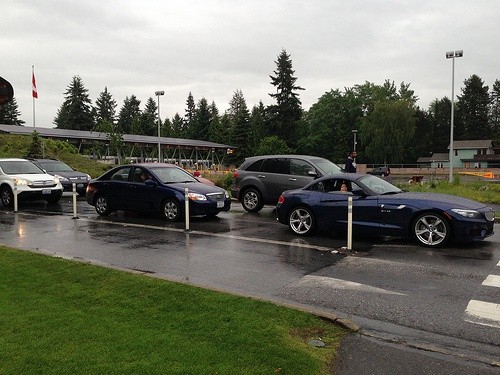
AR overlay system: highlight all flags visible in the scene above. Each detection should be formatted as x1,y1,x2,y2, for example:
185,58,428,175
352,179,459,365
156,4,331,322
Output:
32,73,38,98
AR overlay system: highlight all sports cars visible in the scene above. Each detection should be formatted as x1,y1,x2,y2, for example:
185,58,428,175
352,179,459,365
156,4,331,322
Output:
275,172,495,249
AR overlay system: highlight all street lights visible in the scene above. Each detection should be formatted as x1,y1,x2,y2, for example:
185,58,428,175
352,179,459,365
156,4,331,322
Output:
445,50,464,186
155,91,165,162
352,130,358,162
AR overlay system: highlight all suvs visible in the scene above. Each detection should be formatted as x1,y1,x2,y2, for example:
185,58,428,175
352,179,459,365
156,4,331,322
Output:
231,154,367,214
0,157,63,211
365,167,390,176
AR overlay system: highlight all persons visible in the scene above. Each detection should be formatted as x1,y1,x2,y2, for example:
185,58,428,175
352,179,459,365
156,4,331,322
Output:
345,151,358,173
340,183,347,192
140,172,148,183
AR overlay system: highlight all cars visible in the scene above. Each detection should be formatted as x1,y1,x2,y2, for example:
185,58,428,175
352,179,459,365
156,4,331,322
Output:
86,162,233,222
194,176,216,186
23,158,92,197
